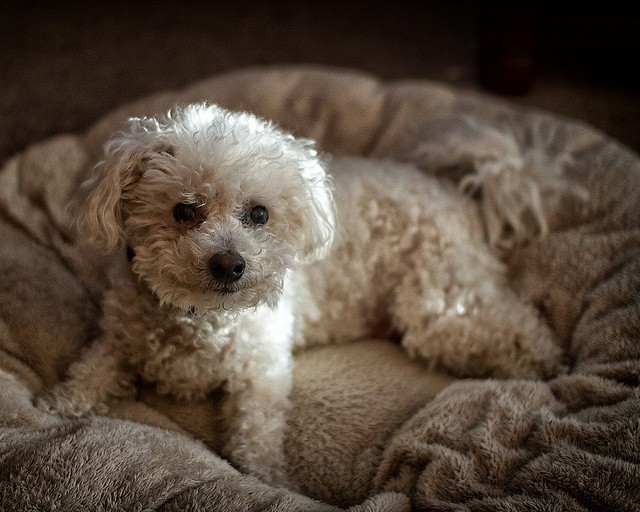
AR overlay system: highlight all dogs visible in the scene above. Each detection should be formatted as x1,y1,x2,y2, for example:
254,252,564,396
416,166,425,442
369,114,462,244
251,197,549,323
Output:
37,99,563,488
